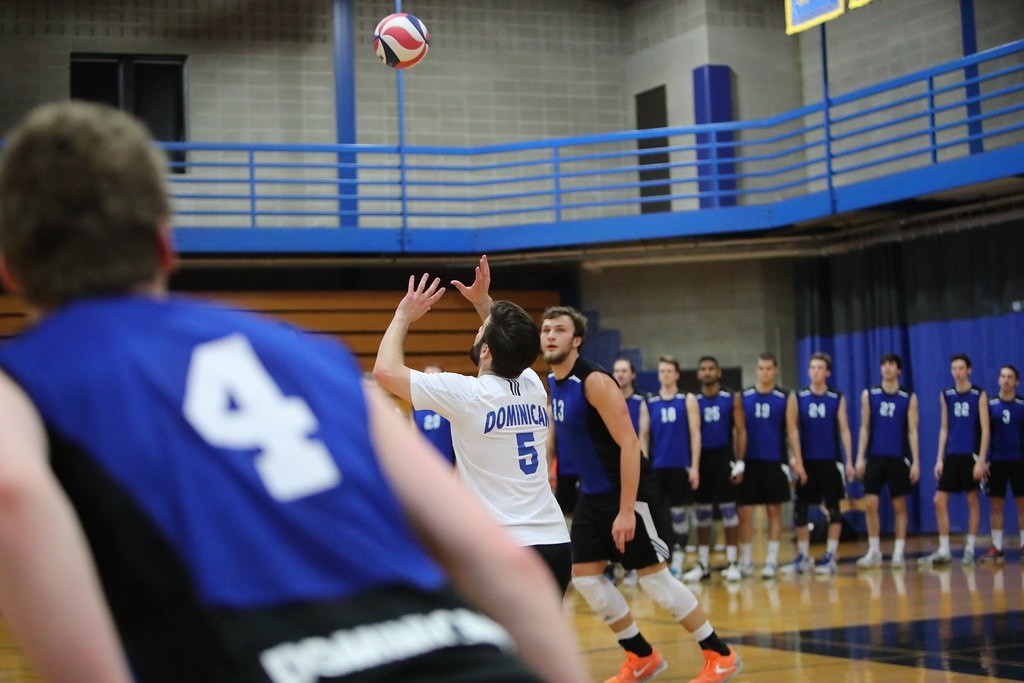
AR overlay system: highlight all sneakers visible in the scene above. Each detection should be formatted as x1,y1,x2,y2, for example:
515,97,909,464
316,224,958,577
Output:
891,550,905,567
960,550,976,566
686,643,743,683
980,545,1005,560
760,559,778,578
917,552,952,566
813,551,839,574
600,643,668,683
855,552,883,567
778,553,810,573
682,561,712,581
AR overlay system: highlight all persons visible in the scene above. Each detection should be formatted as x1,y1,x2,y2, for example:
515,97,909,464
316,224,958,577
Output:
597,561,1024,683
0,98,595,683
917,353,1024,565
371,255,573,610
413,366,456,465
540,306,744,683
603,351,855,588
855,353,921,568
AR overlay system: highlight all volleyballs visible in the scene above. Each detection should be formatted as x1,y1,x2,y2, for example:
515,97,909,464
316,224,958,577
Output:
373,12,431,70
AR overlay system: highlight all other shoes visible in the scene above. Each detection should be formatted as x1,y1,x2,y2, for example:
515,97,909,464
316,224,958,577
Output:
621,570,637,586
670,566,683,579
719,562,754,581
